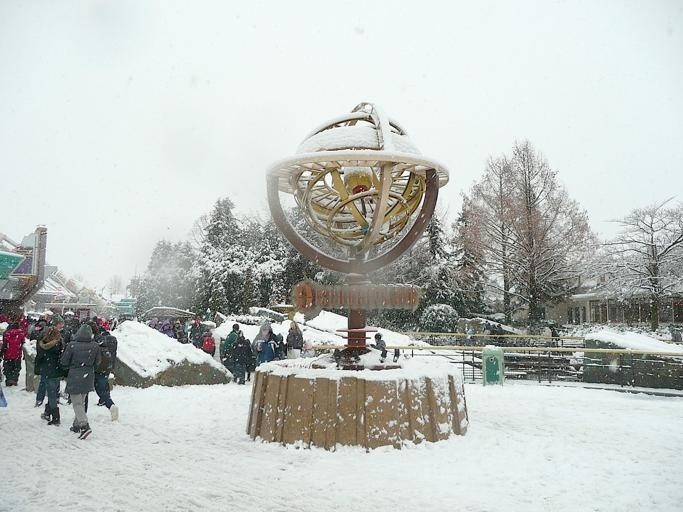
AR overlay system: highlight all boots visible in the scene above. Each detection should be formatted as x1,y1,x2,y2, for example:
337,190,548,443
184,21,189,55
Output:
40,404,60,425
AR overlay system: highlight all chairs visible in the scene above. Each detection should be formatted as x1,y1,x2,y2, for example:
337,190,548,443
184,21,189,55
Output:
461,343,585,385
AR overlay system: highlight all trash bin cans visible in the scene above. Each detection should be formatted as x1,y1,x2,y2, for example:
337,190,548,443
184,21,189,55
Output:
482,345,505,387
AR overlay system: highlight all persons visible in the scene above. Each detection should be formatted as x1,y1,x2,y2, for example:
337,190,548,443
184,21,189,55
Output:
33,327,65,427
483,320,560,346
58,324,102,440
222,321,303,384
51,314,122,344
147,317,216,358
370,333,385,351
0,310,51,392
91,322,118,421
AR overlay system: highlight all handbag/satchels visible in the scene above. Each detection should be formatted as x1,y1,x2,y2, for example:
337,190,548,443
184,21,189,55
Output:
203,336,214,353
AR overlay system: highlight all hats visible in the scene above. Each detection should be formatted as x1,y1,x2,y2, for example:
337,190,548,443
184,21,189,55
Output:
78,324,93,337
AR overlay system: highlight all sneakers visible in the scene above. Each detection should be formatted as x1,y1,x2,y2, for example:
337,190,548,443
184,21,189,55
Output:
111,406,119,421
70,424,92,440
